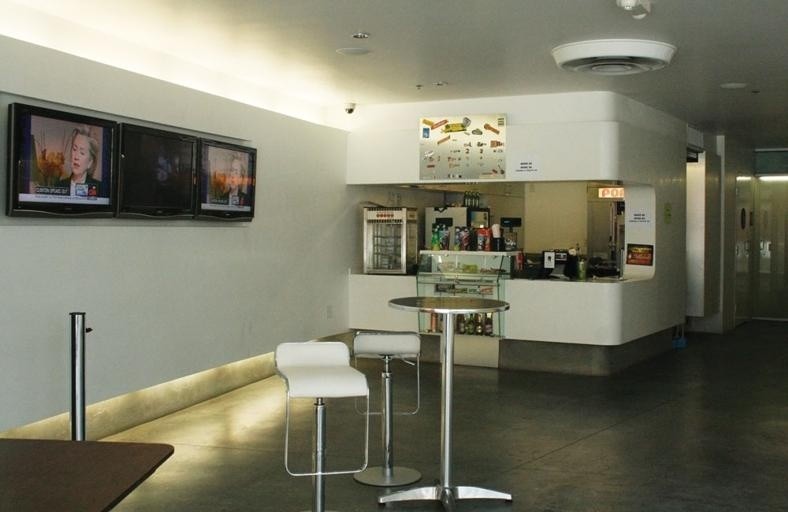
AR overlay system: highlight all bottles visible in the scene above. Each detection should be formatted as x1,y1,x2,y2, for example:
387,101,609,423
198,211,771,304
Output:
431,314,492,335
430,223,504,251
464,191,480,207
577,256,587,282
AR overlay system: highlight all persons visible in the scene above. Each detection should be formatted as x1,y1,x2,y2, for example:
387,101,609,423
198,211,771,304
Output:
53,124,103,198
219,152,251,207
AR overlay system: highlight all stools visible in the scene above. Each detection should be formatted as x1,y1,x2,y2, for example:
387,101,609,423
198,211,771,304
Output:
274,342,370,512
352,332,422,488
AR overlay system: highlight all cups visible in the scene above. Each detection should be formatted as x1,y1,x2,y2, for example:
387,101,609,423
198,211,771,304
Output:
504,232,517,251
511,253,524,276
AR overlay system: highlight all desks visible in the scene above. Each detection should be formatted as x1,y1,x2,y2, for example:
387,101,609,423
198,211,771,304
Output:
377,298,512,512
0,437,174,512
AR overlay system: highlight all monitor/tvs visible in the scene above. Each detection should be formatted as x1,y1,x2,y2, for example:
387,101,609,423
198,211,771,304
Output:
118,122,198,222
8,101,118,222
198,137,256,222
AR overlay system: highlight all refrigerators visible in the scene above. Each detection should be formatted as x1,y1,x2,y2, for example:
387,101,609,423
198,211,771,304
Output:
362,206,417,277
425,207,492,249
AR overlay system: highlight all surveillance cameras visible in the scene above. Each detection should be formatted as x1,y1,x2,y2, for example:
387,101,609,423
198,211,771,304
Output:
344,104,357,114
